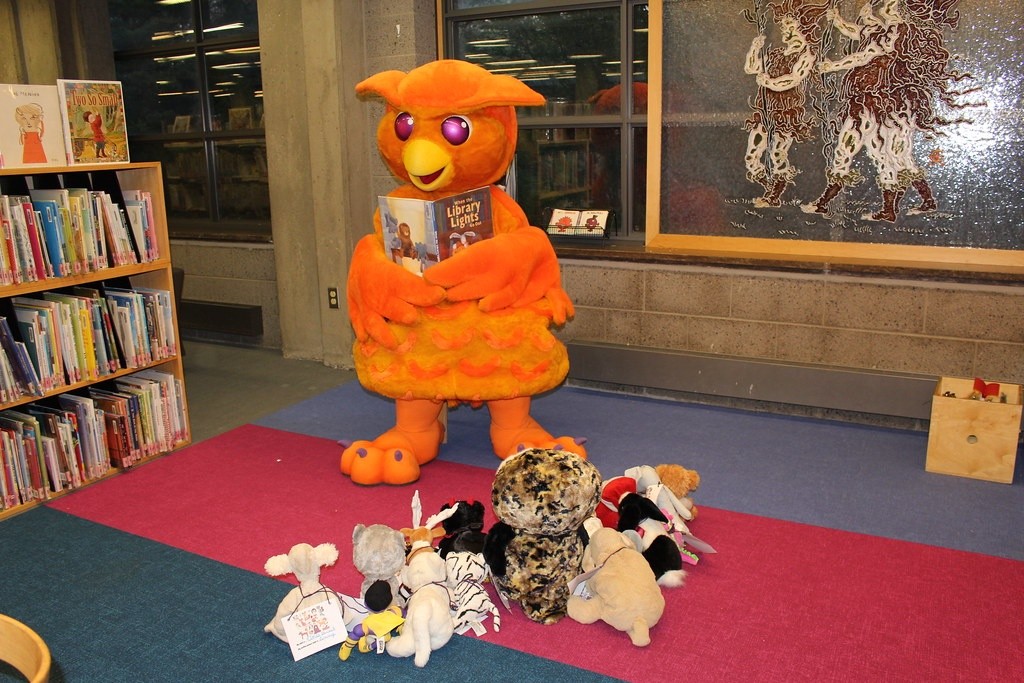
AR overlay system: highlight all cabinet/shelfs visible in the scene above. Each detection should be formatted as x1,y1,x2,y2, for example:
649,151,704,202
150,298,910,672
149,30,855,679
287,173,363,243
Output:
0,161,192,520
504,101,604,224
161,117,270,220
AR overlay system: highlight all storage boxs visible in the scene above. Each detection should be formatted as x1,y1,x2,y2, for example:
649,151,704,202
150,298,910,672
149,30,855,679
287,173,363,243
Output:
924,373,1023,485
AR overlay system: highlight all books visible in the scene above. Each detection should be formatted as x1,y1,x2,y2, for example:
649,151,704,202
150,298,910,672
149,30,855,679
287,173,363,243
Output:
375,183,494,278
0,178,161,291
0,283,179,407
54,77,132,165
0,366,191,517
576,210,609,237
547,208,580,238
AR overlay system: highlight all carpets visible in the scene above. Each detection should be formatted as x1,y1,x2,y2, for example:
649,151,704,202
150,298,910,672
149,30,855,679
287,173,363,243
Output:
0,375,1024,683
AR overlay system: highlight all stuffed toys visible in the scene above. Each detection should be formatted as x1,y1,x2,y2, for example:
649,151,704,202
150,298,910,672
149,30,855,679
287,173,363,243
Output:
263,447,722,669
337,53,590,488
